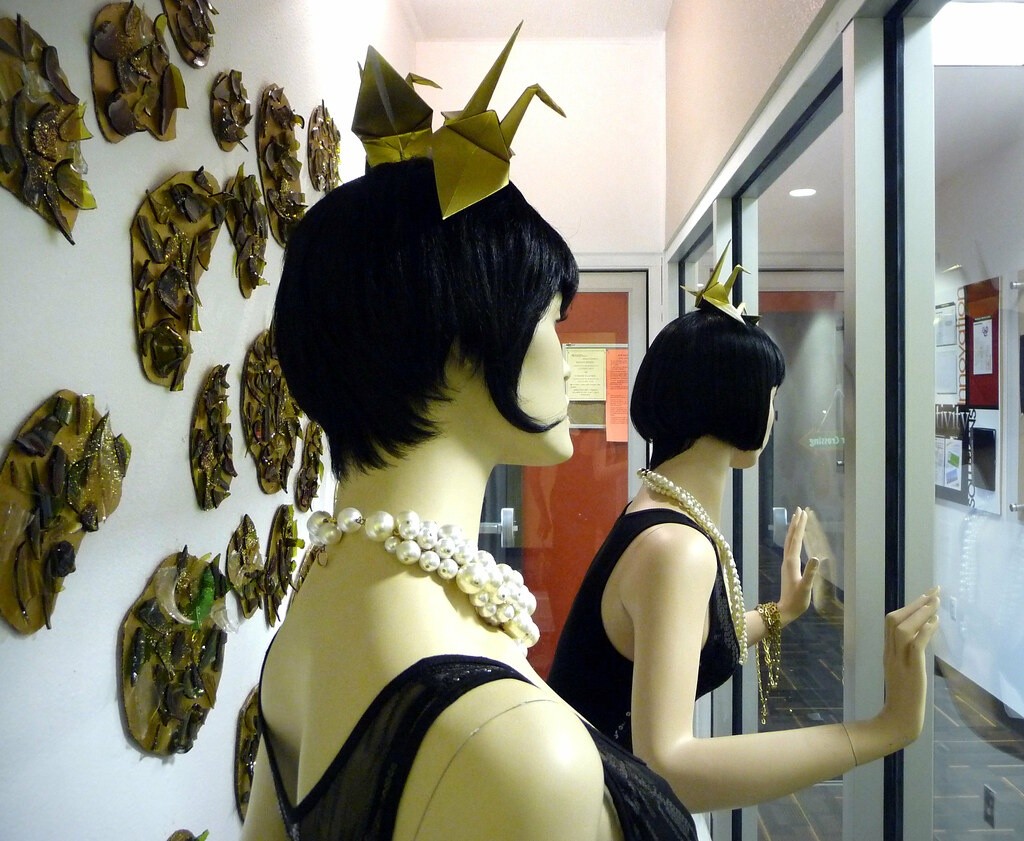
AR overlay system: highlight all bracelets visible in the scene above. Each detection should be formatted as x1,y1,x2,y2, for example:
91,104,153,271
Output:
749,605,781,727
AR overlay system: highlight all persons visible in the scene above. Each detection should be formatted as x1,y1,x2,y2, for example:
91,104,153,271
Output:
549,312,943,814
230,156,697,841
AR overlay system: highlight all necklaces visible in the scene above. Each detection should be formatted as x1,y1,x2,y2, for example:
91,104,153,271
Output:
637,467,748,665
303,509,539,647
